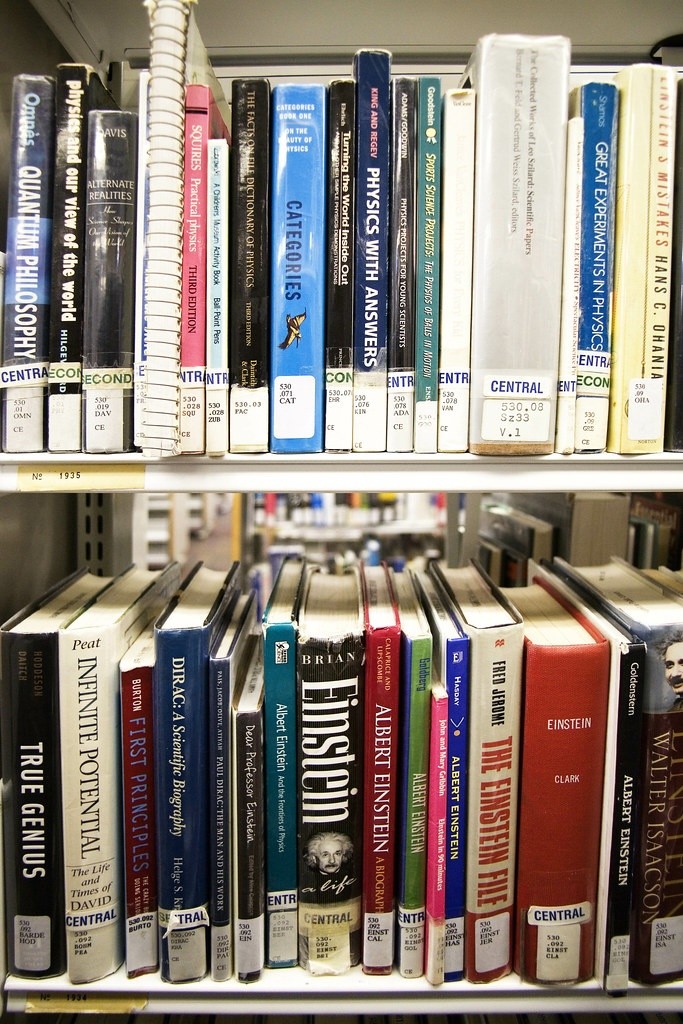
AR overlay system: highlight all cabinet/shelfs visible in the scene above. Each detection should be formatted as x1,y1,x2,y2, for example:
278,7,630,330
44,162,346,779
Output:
1,0,683,1024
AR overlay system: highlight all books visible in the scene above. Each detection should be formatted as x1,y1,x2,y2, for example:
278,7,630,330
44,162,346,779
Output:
0,0,683,1024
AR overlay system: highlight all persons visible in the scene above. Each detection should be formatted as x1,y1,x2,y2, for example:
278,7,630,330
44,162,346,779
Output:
655,630,683,713
303,831,354,903
